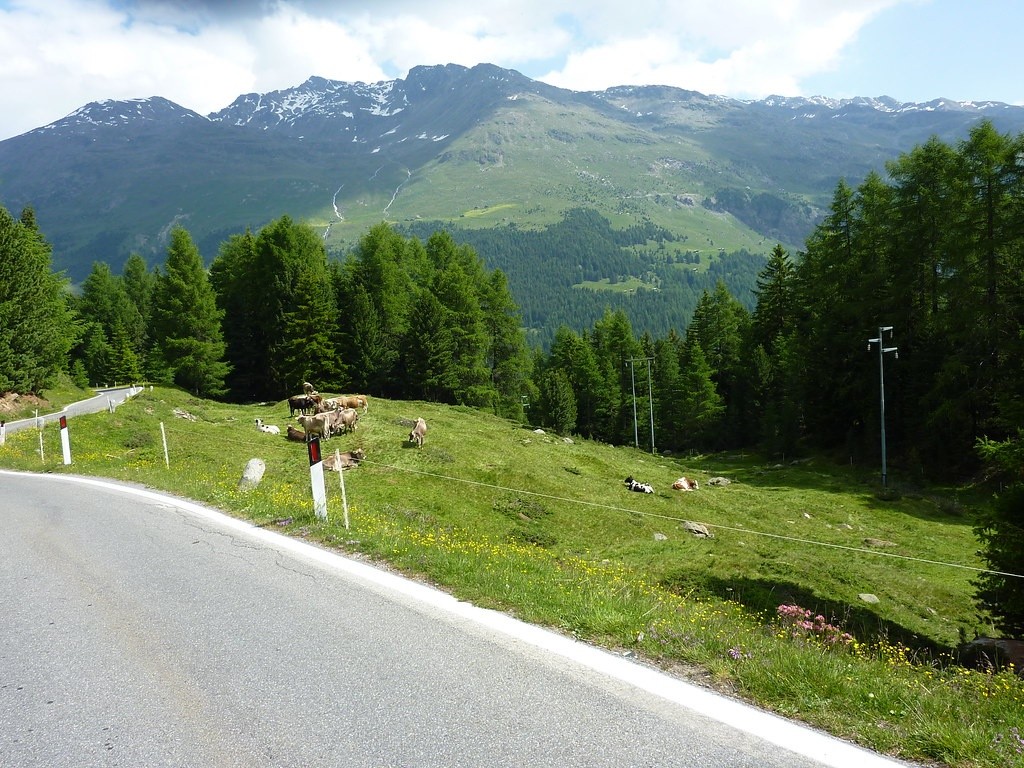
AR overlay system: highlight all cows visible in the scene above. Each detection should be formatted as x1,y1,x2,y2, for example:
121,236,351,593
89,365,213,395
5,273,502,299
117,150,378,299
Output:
322,447,367,472
284,381,368,443
254,418,281,435
671,477,699,491
624,475,655,494
408,417,427,448
